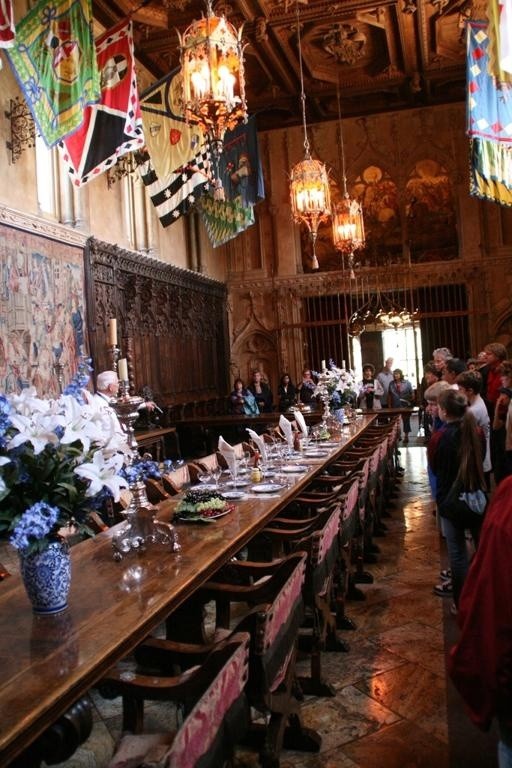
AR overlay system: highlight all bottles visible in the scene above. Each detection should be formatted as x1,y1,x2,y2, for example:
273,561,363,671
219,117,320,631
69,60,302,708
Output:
310,426,320,441
294,432,300,451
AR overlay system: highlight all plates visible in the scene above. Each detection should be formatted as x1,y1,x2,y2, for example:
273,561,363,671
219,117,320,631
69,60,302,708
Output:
179,508,231,522
190,440,338,500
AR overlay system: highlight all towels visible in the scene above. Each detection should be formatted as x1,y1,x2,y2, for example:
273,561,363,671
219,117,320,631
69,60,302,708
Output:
217,406,313,481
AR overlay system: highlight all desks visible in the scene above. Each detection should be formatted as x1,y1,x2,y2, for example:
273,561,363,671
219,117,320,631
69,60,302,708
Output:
131,427,176,461
168,406,421,460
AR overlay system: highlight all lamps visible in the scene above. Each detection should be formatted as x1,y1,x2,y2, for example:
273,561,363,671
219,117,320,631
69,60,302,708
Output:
172,1,251,200
328,80,370,282
288,12,336,272
347,291,422,339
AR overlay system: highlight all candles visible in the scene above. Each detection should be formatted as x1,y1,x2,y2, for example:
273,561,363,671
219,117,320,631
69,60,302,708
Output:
117,357,129,382
107,318,118,347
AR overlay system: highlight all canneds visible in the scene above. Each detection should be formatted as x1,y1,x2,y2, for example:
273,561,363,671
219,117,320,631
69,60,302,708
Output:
250,468,260,482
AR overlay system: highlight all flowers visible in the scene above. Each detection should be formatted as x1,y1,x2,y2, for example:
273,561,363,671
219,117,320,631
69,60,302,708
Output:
1,381,134,546
305,368,360,406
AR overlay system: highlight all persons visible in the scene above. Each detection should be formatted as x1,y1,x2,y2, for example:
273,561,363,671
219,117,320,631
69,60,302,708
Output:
447,472,512,768
230,368,320,416
417,342,511,594
93,366,160,467
356,358,415,444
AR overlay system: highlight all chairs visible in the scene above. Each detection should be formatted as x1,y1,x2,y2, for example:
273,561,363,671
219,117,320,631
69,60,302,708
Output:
110,479,172,522
161,463,207,494
52,507,108,542
192,416,404,679
137,551,323,765
87,631,251,768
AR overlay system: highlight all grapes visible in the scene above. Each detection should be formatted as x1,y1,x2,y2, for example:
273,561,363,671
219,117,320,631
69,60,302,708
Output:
172,487,236,519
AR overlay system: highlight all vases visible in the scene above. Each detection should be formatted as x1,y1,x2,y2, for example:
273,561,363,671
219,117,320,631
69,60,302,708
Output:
17,537,74,616
330,401,344,429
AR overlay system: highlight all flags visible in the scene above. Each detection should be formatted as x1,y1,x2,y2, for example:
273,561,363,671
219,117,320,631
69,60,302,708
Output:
0,0,18,48
127,66,224,230
57,11,148,191
192,79,265,250
7,0,104,147
462,17,511,208
